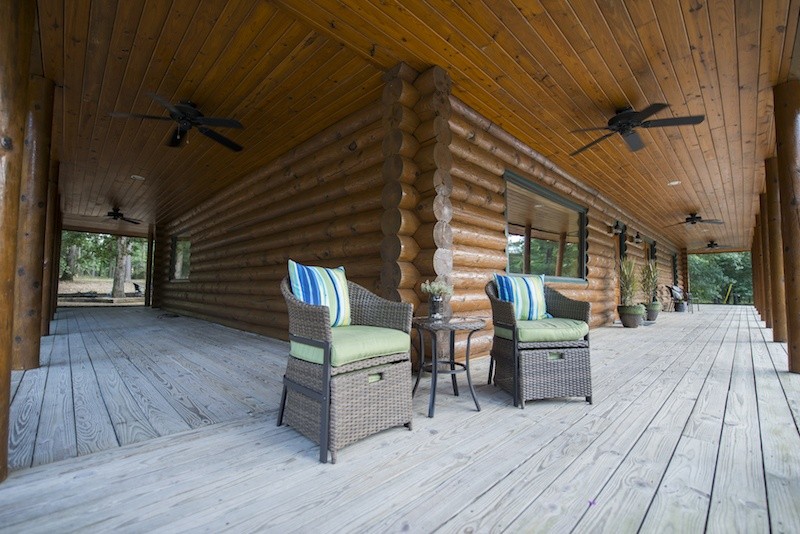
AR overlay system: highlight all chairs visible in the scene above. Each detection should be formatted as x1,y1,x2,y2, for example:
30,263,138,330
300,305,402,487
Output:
277,274,414,463
666,285,699,314
486,280,593,408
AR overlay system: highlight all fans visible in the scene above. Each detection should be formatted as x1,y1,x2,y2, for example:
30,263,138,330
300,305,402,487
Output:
104,208,142,224
664,212,725,228
110,89,245,152
698,240,735,249
569,103,706,156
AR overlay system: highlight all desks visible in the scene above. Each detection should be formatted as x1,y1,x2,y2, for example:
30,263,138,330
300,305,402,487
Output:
411,316,487,417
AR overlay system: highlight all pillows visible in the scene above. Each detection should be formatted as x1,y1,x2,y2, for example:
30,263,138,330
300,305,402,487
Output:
493,272,553,321
288,259,351,326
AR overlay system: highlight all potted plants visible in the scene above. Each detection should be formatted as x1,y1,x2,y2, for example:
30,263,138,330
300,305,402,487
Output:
615,255,662,328
422,280,454,318
